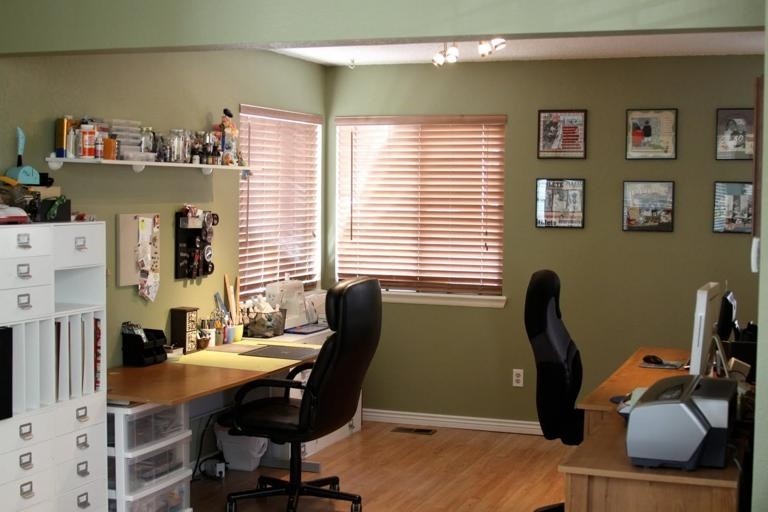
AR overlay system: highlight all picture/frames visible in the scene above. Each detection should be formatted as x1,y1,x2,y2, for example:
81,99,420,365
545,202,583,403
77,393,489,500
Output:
713,181,754,234
622,180,674,233
535,178,585,230
716,108,754,160
625,108,677,160
538,110,586,160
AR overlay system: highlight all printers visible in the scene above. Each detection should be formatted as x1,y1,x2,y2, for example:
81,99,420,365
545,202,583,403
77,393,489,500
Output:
627,374,737,471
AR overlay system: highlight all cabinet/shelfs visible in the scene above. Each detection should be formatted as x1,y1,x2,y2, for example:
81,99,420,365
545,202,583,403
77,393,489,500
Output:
0,222,108,512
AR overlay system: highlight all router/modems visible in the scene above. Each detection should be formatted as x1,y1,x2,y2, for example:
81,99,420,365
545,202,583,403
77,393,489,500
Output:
726,356,751,380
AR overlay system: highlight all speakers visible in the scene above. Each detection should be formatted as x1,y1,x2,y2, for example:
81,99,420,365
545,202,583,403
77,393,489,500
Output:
717,341,756,382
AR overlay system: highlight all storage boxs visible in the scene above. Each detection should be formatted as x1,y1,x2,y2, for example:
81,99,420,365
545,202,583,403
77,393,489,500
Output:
106,403,193,511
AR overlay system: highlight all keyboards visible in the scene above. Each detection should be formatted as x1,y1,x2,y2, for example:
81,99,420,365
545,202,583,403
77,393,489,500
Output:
617,386,648,420
684,357,691,368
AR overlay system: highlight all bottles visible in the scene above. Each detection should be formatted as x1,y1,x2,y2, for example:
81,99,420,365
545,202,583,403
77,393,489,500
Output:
67,118,118,160
142,126,222,165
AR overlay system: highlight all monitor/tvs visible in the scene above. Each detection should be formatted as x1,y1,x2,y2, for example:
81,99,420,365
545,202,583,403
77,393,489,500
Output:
717,289,741,341
689,282,732,378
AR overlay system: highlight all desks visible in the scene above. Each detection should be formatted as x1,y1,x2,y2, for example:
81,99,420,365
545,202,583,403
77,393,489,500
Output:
557,422,741,512
575,347,754,421
109,313,326,404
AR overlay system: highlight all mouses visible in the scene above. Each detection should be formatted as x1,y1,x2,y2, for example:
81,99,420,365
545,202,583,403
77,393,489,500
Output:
643,353,663,365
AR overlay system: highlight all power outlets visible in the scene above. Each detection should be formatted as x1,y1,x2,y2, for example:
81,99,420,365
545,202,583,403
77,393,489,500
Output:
512,368,524,388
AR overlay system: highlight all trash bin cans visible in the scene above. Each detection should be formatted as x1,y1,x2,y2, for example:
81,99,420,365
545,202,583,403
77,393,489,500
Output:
213,424,268,472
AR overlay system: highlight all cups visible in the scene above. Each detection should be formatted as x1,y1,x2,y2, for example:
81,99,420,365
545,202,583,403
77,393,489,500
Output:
233,324,244,341
224,325,234,343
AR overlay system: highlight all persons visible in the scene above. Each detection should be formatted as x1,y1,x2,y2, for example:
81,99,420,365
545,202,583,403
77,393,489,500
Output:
723,118,746,152
642,121,652,147
544,113,562,151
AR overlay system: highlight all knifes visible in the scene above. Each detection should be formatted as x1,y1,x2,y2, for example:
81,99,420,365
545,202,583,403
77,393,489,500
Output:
16,127,25,166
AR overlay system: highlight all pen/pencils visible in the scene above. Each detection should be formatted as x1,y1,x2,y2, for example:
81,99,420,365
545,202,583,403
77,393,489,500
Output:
223,312,235,327
210,310,223,328
202,320,213,328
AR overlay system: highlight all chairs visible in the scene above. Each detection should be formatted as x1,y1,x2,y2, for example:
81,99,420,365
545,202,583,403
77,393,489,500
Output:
213,277,383,512
523,269,584,512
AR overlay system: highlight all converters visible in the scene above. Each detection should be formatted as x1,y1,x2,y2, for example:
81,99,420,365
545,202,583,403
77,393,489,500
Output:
206,462,225,478
